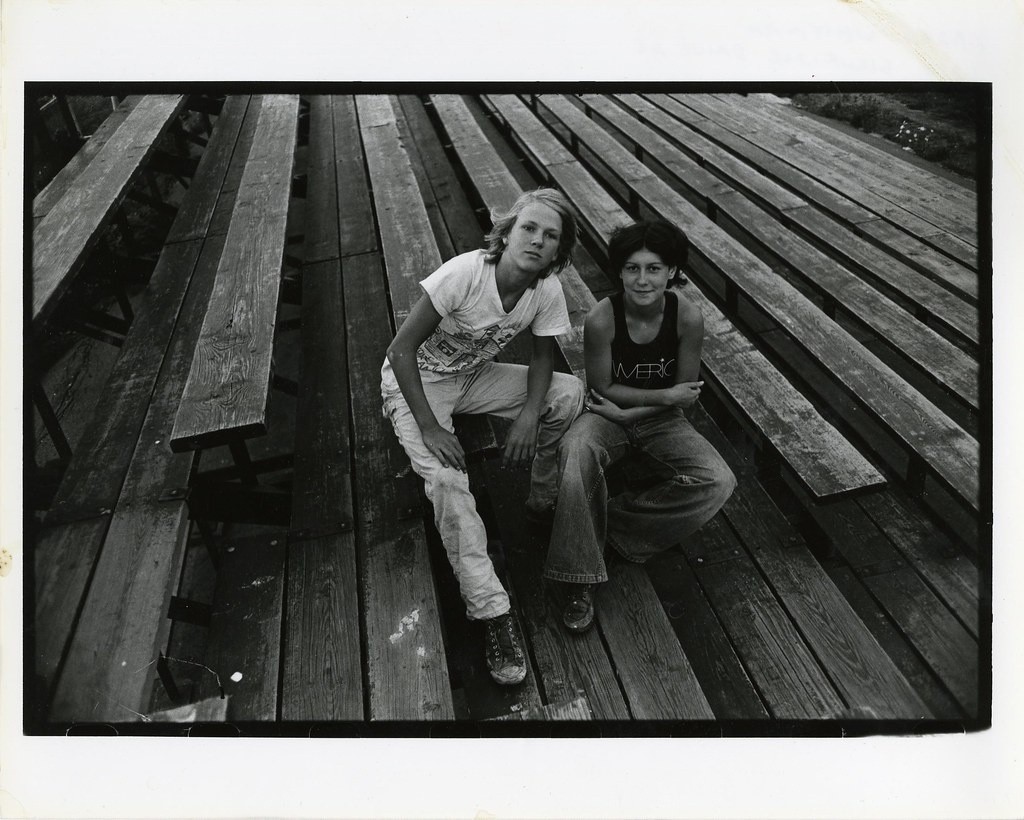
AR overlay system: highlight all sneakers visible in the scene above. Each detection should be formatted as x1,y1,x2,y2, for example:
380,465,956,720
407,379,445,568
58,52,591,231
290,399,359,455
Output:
603,545,625,571
563,579,596,632
485,616,527,685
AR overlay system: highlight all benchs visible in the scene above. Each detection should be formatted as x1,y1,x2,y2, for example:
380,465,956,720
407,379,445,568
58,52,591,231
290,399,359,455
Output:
28,92,979,718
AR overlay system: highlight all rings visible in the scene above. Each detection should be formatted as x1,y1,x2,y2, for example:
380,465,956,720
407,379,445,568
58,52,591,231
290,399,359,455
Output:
586,403,592,410
598,396,604,401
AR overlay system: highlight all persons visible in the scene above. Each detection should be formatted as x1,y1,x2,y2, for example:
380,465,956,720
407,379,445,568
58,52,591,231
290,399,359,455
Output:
544,217,739,637
380,188,585,686
